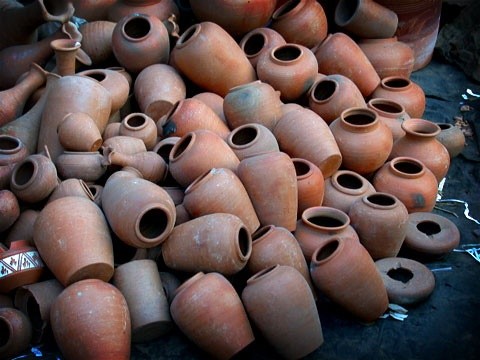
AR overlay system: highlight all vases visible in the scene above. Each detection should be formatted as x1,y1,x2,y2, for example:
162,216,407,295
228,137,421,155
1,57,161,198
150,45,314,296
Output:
0,0,465,360
